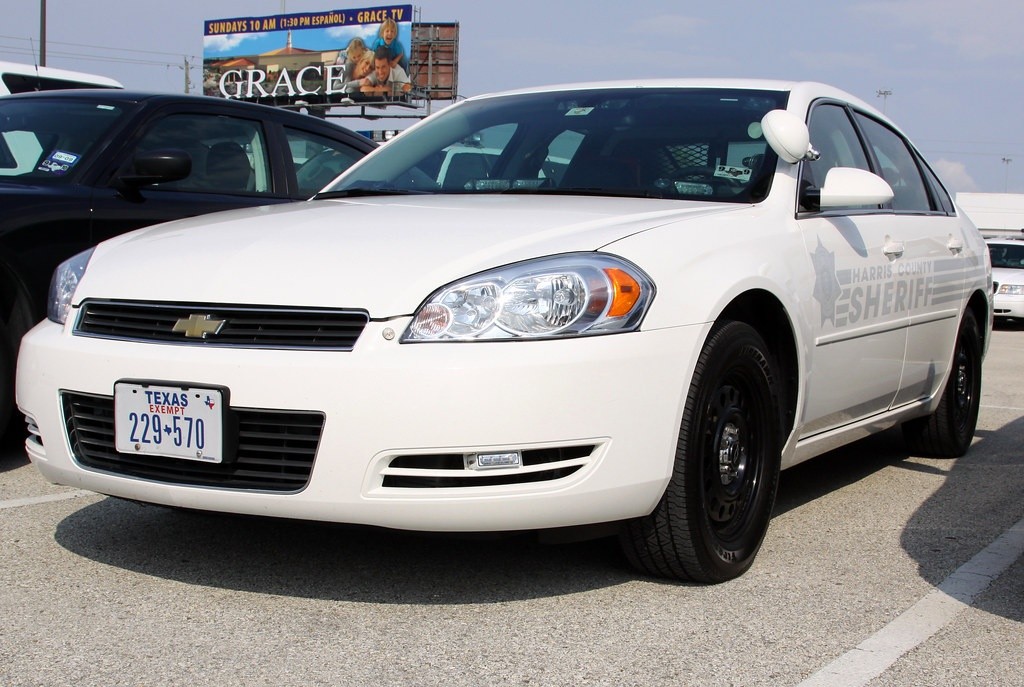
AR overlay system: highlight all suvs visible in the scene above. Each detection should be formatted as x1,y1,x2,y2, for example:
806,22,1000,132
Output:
0,59,126,179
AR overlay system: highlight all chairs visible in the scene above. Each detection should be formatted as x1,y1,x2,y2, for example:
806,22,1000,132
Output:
610,138,682,194
991,250,1002,261
206,142,251,191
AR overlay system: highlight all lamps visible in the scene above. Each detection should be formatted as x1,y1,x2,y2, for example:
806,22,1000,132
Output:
341,98,354,103
295,100,308,106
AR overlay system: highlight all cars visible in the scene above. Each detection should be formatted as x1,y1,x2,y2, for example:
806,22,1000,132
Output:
292,139,573,196
980,235,1024,318
0,88,444,436
15,76,995,586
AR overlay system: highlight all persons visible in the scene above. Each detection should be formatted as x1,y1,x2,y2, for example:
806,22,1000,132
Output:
330,17,412,103
